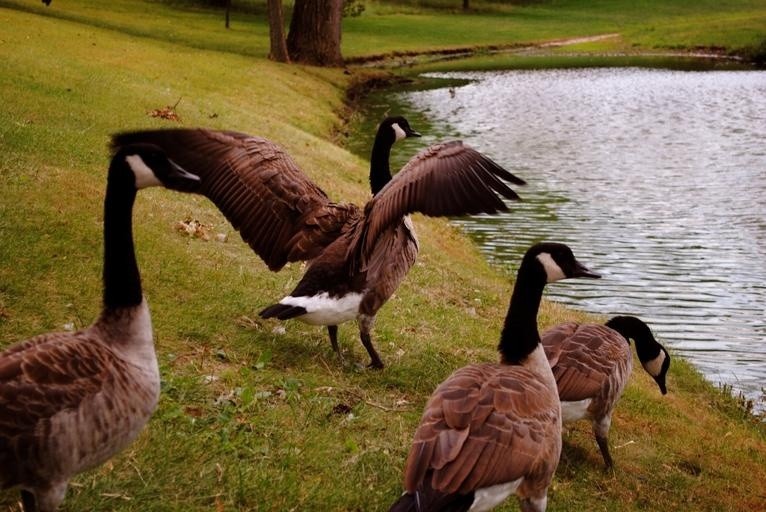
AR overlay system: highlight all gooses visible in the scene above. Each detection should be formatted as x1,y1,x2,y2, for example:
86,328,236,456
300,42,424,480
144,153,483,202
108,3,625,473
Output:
387,241,603,512
539,315,671,477
0,143,202,512
106,114,528,371
